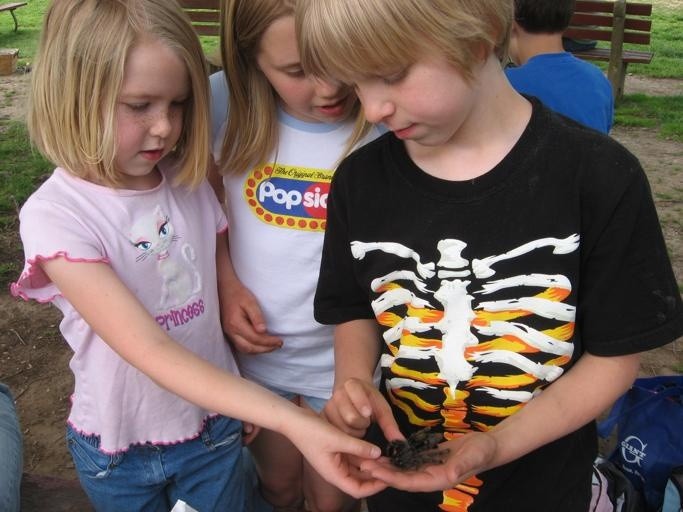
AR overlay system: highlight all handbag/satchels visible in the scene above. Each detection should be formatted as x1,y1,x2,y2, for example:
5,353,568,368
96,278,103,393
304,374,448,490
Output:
588,376,683,511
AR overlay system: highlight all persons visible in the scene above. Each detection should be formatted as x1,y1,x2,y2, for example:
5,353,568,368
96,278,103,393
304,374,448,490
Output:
8,0,390,512
215,0,421,512
499,0,615,136
294,0,682,512
